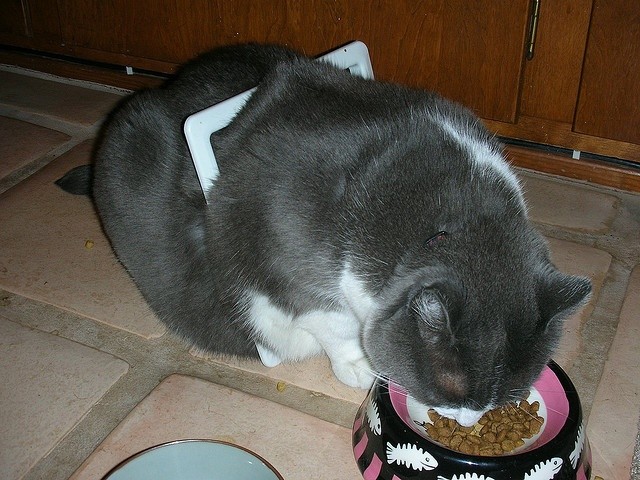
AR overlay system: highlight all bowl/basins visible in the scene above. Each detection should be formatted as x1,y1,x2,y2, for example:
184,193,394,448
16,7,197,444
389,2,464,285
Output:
351,357,592,480
98,438,285,480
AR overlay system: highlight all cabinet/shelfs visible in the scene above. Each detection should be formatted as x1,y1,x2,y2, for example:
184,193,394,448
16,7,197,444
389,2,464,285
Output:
574,1,639,193
2,1,542,171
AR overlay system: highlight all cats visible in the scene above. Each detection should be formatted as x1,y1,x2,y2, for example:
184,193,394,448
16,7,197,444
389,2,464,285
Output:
52,41,596,427
